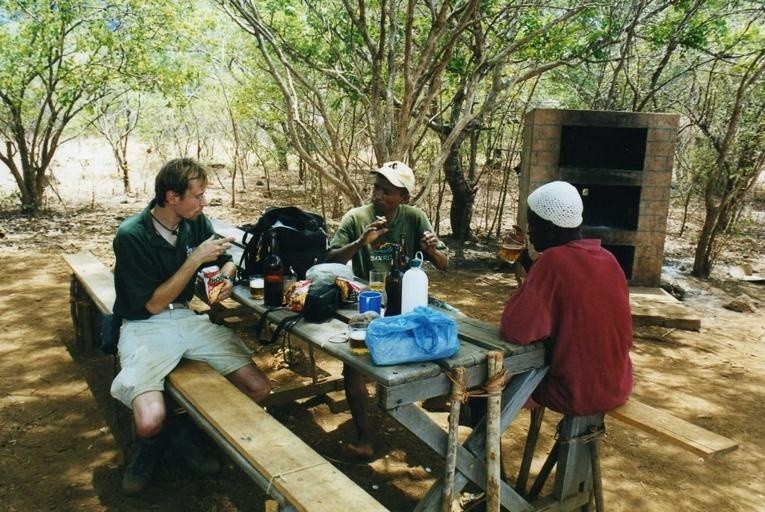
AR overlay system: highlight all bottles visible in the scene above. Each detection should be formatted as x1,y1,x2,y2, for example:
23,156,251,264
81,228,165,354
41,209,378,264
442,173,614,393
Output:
384,243,404,317
261,232,283,307
390,233,410,275
401,251,428,315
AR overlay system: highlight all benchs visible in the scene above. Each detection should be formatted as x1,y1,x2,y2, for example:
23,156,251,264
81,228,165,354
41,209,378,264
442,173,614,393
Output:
61,252,393,512
552,387,734,504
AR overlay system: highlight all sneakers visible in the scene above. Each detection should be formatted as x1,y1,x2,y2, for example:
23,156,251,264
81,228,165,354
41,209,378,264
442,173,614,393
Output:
121,440,160,497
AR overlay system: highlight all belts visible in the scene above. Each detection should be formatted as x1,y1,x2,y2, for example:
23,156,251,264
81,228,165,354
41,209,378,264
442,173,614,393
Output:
166,300,189,309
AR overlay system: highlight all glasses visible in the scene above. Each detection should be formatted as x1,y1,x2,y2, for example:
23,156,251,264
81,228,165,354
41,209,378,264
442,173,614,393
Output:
187,194,205,202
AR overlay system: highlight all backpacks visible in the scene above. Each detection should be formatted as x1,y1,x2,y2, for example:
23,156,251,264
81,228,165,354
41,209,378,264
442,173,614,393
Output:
230,206,329,285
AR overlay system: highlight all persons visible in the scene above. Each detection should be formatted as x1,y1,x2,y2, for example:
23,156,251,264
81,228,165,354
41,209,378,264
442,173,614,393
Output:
449,181,632,434
111,157,272,495
325,162,450,466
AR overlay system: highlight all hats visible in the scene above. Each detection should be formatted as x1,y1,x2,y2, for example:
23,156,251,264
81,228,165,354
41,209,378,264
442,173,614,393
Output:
527,181,584,228
369,160,416,194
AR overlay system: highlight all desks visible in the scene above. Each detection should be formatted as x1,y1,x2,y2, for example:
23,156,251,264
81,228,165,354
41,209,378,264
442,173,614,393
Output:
184,212,552,512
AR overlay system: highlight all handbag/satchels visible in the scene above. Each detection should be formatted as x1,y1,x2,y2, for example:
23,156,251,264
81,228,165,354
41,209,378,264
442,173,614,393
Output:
254,286,340,346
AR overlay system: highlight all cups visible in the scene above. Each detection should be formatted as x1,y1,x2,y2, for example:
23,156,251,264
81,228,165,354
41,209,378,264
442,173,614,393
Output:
498,233,526,265
349,322,371,354
359,293,381,318
283,273,297,296
369,270,387,294
248,274,266,300
